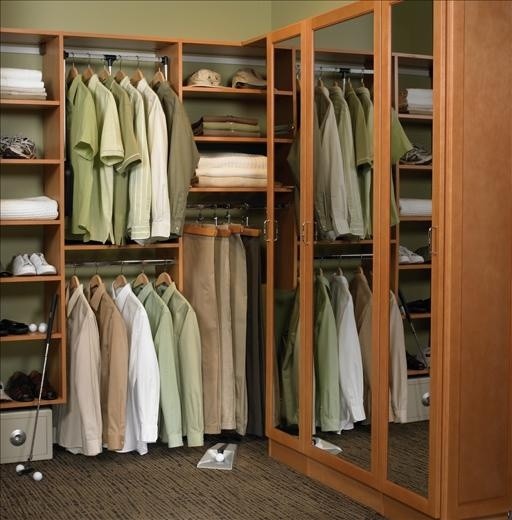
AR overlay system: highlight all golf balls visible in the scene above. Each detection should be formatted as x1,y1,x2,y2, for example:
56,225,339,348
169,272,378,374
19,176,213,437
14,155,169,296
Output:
27,322,49,334
215,454,224,460
14,465,26,474
33,472,42,481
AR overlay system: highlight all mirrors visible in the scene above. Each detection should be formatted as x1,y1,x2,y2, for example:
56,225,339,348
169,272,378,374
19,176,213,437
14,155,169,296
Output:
311,7,375,475
270,32,301,441
386,0,434,502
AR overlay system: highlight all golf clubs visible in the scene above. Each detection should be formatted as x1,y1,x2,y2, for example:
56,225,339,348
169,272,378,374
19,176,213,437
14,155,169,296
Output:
394,284,429,406
18,293,58,475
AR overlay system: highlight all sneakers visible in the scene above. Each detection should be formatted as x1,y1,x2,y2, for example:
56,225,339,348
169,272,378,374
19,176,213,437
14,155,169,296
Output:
403,246,424,264
4,371,34,402
0,321,8,337
399,245,411,265
406,299,429,313
28,370,57,400
29,252,57,275
12,253,37,276
406,350,424,370
1,319,29,335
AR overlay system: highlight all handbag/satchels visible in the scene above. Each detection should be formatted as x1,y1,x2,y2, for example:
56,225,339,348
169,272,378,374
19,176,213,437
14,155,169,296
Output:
0,136,37,160
399,142,433,165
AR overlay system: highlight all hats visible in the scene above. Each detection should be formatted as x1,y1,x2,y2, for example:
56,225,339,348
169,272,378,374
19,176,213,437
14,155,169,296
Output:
232,68,267,88
187,69,224,88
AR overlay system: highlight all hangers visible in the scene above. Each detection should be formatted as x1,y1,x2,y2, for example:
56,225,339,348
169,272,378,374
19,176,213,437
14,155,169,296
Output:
66,257,172,291
183,201,262,240
296,64,366,90
316,252,374,278
66,50,166,87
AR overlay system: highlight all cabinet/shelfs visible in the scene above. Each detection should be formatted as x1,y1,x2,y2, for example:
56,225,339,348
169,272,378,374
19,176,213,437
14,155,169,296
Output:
273,44,433,379
0,26,267,412
